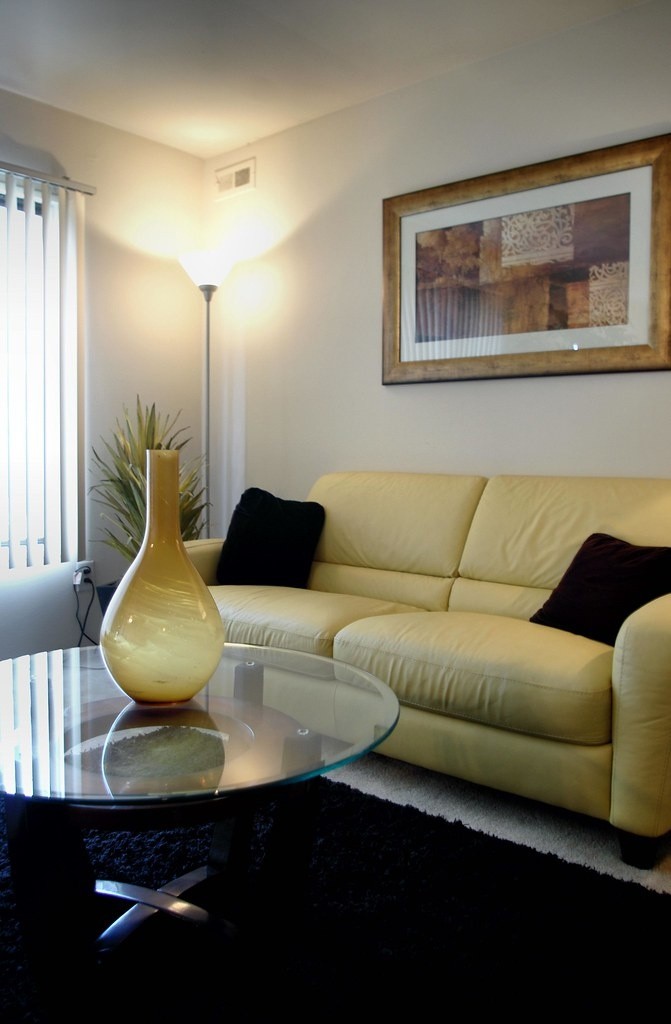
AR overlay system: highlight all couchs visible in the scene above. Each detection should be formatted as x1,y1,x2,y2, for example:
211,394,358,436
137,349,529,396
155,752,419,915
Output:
182,471,671,869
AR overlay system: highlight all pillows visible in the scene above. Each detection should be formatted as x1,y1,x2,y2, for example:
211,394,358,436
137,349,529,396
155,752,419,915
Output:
529,533,671,647
216,488,325,590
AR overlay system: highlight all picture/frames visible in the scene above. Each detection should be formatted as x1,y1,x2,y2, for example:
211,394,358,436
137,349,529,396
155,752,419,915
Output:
381,132,670,386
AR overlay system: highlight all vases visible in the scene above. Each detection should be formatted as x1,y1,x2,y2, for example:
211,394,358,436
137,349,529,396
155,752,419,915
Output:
98,448,224,704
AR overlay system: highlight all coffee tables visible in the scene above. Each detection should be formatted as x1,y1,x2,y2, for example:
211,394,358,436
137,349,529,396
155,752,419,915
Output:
0,642,399,953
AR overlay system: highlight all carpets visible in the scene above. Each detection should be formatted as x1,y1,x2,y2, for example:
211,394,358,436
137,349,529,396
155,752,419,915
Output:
319,750,671,894
0,725,671,1024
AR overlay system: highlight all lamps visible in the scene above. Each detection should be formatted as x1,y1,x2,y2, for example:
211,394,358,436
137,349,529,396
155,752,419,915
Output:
176,253,234,538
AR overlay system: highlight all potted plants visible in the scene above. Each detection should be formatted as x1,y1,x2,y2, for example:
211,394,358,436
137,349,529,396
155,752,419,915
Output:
85,393,213,617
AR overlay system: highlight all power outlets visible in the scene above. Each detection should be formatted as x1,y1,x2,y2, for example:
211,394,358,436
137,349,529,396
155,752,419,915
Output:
75,561,97,593
